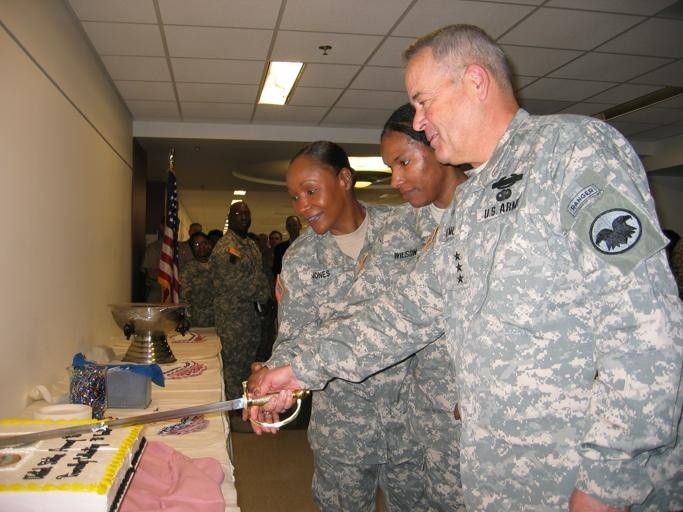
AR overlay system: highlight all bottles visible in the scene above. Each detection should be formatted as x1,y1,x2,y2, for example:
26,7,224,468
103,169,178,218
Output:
254,302,270,315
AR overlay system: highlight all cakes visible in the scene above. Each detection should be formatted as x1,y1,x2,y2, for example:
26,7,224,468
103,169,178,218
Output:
0,418,147,512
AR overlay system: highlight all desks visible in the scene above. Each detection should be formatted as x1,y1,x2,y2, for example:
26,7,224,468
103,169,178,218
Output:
0,319,237,511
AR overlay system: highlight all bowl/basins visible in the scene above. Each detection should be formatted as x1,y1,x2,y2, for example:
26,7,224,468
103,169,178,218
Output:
108,304,188,337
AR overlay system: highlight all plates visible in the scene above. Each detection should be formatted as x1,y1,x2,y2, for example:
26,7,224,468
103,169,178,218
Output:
33,403,93,421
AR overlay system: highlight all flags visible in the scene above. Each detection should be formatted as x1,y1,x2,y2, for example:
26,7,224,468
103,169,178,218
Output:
155,164,182,303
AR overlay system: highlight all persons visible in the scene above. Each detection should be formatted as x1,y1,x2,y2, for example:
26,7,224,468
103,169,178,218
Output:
248,99,473,512
255,24,682,512
243,141,426,512
141,201,303,417
